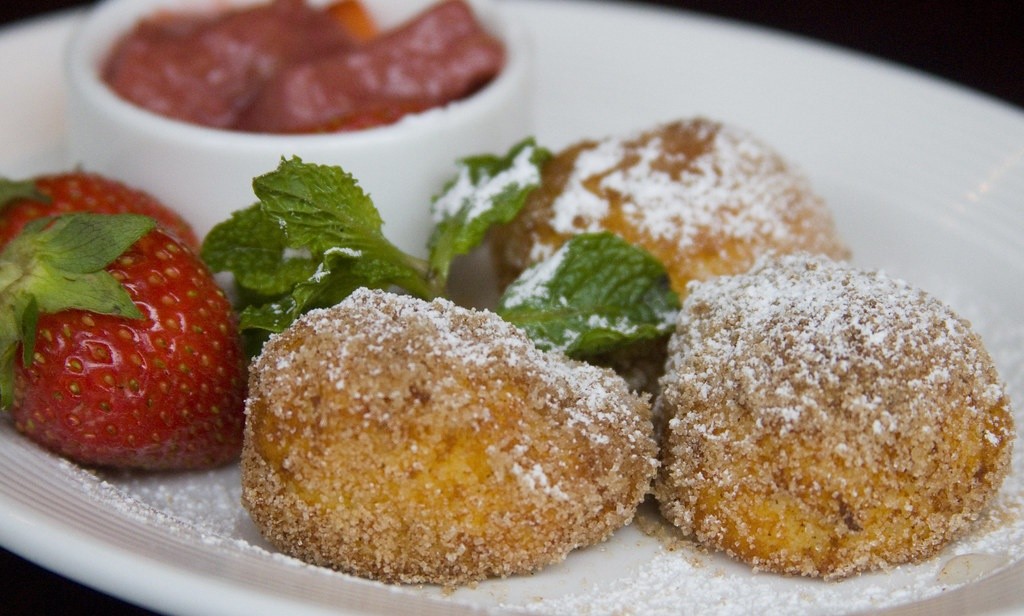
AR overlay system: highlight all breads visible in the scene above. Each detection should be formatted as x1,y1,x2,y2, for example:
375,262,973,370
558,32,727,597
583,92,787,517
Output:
239,285,659,586
485,114,853,310
641,257,1014,582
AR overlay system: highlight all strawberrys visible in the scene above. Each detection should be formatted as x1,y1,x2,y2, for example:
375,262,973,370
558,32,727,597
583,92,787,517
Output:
0,172,249,474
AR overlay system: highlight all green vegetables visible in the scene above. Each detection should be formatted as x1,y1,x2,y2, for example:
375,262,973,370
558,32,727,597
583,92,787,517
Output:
188,132,685,360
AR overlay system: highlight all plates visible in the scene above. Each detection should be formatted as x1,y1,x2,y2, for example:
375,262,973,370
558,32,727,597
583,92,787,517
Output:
0,1,1024,615
